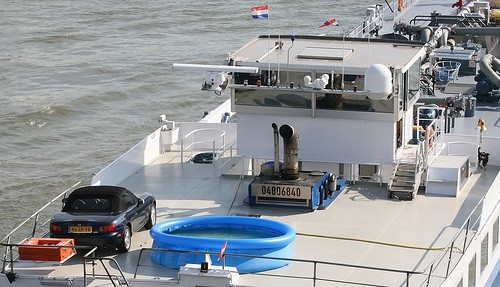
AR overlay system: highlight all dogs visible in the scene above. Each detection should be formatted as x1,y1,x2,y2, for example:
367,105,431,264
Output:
478,146,490,171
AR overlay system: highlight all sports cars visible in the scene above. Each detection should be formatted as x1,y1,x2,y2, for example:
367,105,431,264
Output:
49,186,156,253
